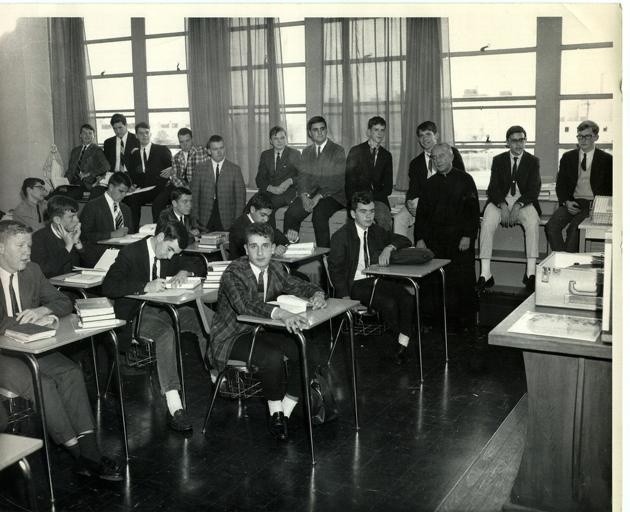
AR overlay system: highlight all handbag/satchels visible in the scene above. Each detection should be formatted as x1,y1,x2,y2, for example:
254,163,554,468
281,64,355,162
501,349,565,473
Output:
390,248,433,264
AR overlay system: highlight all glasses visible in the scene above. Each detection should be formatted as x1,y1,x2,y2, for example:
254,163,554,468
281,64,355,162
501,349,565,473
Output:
577,134,592,139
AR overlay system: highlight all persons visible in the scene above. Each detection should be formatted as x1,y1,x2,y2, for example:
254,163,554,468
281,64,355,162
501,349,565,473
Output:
473,126,542,294
544,121,612,253
3,112,481,482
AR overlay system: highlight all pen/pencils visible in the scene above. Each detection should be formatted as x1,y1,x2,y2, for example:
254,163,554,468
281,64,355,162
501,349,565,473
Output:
275,243,288,258
76,270,106,276
155,275,168,289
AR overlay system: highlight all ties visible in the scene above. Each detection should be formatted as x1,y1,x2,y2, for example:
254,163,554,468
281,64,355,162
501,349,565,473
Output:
215,164,219,192
364,231,368,268
511,157,518,195
276,153,280,171
581,153,586,170
259,270,264,303
9,274,19,316
152,257,157,280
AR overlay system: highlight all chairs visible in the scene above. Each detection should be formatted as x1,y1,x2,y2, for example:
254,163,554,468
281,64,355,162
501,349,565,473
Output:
193,290,292,442
101,299,165,401
320,255,406,370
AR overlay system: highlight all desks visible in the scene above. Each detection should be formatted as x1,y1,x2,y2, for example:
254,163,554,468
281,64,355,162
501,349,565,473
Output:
43,266,107,309
359,258,453,384
178,230,232,262
129,281,224,417
2,311,132,505
264,241,332,282
95,231,152,253
235,293,365,466
0,429,49,511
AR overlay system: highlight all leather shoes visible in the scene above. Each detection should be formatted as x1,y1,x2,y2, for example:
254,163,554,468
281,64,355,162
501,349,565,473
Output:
476,276,494,288
166,409,192,430
523,273,535,290
271,411,289,441
78,455,123,481
